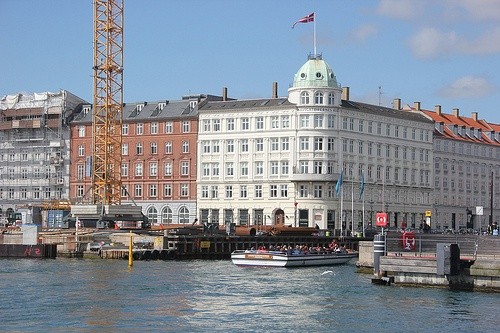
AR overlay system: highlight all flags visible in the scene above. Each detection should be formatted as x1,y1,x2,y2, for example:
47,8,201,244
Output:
358,175,364,200
335,172,343,193
291,12,314,29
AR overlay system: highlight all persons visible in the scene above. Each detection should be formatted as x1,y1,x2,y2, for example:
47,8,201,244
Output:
378,217,385,224
400,220,405,232
244,239,347,254
418,219,432,234
486,222,498,235
315,224,319,229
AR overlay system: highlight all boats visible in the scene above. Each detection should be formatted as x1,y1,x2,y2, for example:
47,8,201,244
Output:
230,249,359,267
